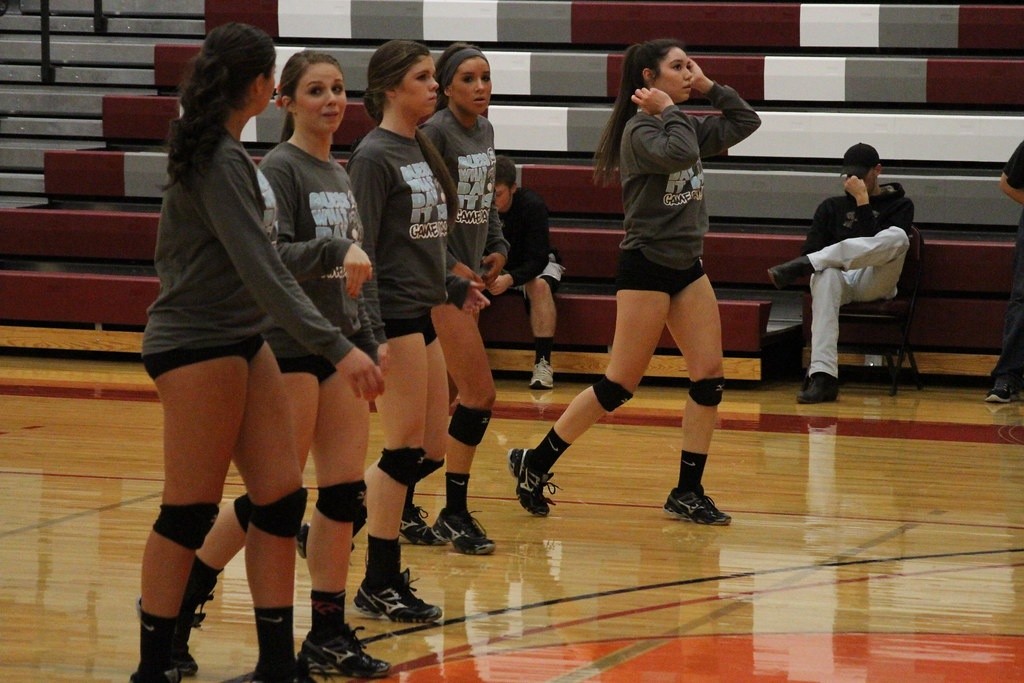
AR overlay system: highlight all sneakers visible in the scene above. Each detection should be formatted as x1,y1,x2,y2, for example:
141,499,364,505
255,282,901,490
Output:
663,485,731,525
173,636,198,675
397,505,447,546
797,378,838,404
296,522,310,559
507,447,564,517
767,262,797,289
431,506,496,556
353,567,442,622
985,380,1011,402
296,622,392,678
528,355,553,388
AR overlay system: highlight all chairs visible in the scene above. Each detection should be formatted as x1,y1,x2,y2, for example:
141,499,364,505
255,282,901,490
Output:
800,225,925,397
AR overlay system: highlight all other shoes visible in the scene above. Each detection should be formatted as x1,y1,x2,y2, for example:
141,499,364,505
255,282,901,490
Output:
129,667,182,683
250,660,318,683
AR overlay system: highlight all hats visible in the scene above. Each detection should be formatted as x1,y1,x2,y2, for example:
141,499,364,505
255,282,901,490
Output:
840,143,878,178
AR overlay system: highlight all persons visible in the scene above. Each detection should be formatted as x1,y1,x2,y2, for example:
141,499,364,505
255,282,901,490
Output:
488,158,566,388
131,23,388,683
768,142,914,403
985,141,1024,402
174,52,392,678
509,39,761,526
301,41,491,623
399,44,511,555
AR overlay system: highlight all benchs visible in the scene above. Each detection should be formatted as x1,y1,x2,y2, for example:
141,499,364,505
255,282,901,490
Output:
0,0,1024,356
